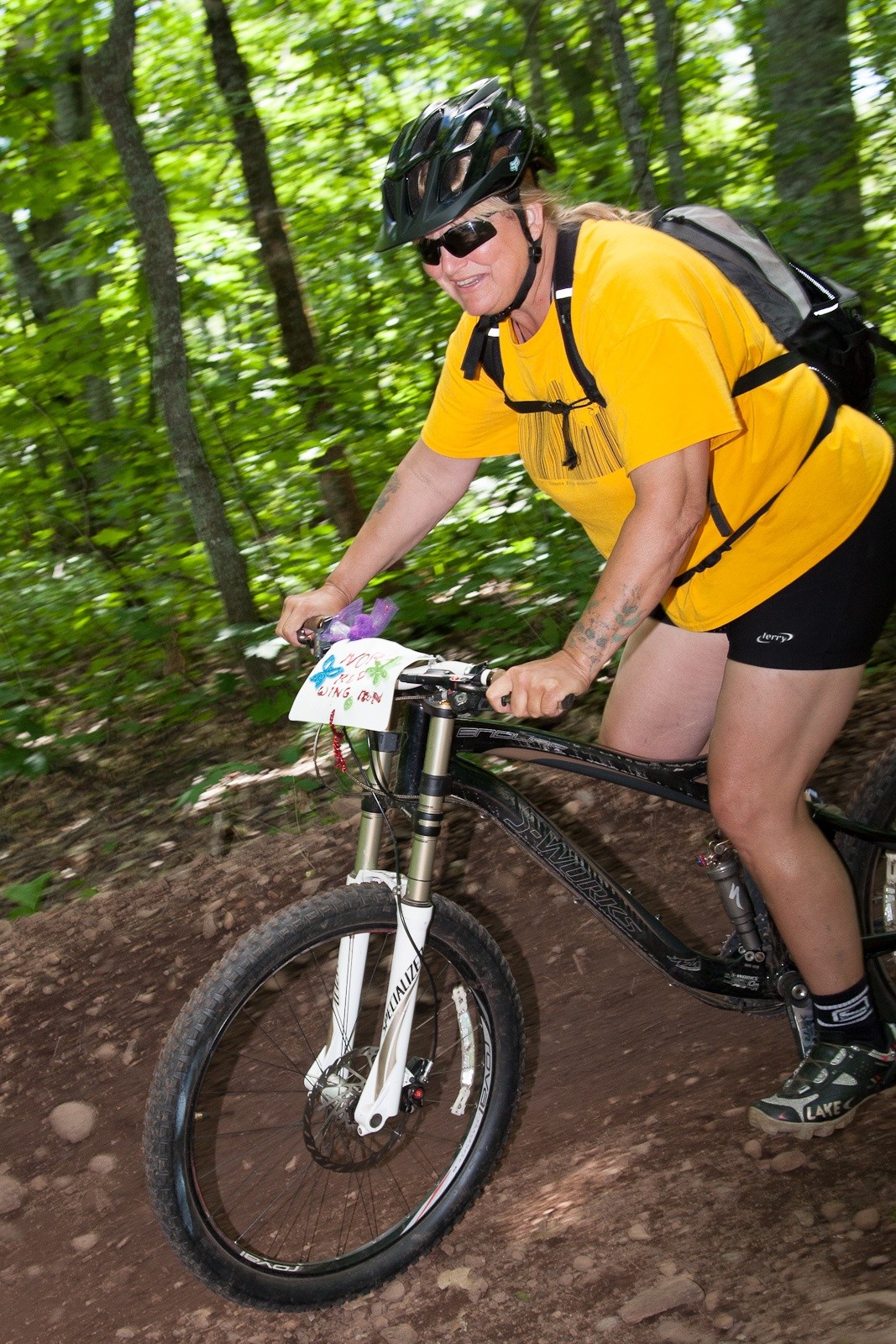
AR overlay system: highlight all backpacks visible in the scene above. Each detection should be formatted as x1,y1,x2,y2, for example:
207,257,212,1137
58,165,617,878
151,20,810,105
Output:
459,198,896,471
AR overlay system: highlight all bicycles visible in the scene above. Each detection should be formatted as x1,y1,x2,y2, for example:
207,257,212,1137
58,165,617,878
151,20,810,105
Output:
139,610,896,1310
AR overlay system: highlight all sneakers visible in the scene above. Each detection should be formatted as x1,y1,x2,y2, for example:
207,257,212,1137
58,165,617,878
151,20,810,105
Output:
745,1022,895,1142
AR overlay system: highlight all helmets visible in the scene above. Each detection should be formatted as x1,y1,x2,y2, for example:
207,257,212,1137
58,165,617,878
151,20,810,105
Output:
369,73,539,255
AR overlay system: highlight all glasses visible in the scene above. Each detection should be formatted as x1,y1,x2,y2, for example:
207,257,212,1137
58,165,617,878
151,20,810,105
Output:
412,203,515,266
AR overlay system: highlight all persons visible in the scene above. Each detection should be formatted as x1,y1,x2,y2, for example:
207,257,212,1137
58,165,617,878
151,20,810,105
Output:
275,76,896,1140
